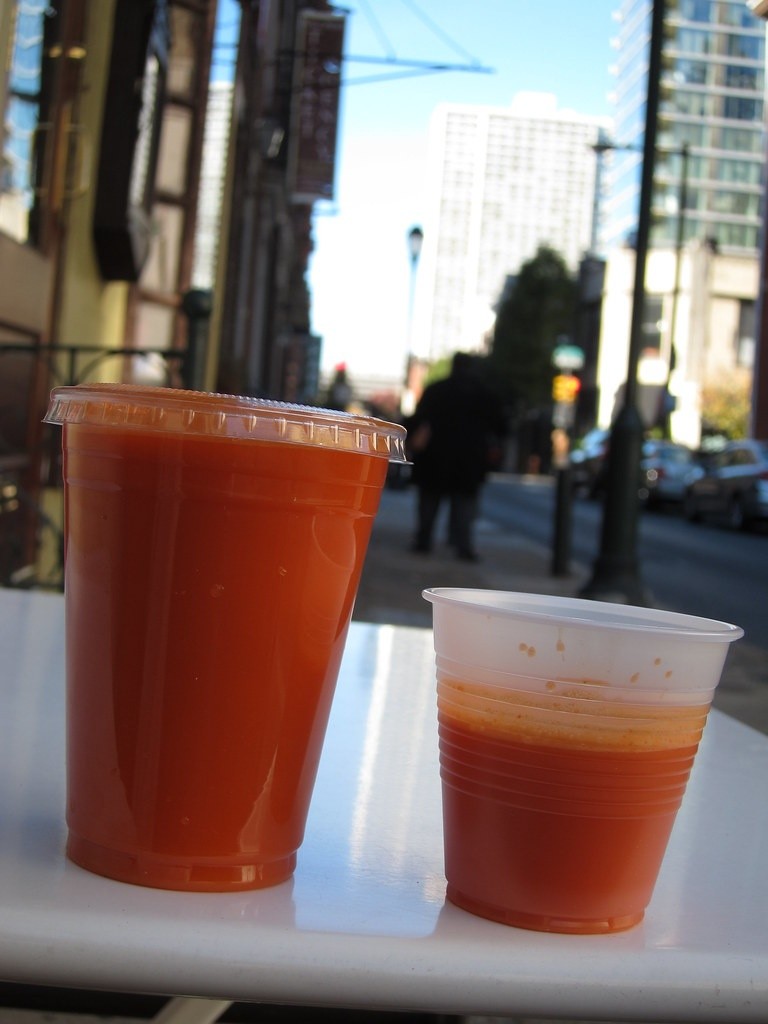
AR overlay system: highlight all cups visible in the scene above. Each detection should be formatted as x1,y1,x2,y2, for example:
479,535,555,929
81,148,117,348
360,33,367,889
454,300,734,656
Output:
424,583,745,936
41,378,410,898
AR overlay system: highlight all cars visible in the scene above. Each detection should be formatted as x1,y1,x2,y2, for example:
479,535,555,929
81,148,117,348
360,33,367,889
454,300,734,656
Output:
569,429,768,535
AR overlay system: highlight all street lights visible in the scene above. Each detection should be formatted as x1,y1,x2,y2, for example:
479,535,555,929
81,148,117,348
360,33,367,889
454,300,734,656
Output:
403,226,424,350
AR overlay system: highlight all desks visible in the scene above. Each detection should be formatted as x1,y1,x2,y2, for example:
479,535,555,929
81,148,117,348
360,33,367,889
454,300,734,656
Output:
0,588,767,1023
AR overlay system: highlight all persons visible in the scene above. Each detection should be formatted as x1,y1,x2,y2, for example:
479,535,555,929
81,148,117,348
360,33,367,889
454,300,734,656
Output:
329,369,351,409
406,352,494,555
